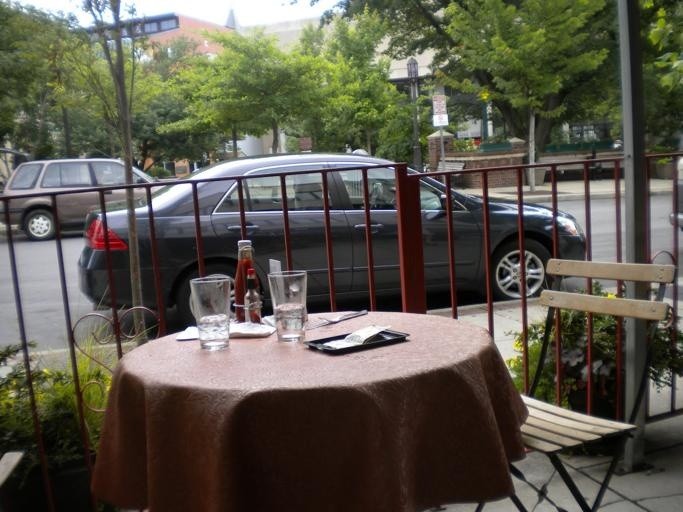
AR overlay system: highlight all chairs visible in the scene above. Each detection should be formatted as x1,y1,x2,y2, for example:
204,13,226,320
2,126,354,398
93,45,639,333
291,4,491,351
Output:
473,259,677,512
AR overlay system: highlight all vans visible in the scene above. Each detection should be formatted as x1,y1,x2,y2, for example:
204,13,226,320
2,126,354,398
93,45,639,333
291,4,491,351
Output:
1,158,167,241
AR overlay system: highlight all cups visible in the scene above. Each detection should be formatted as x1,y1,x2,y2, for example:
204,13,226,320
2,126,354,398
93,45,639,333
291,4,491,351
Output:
189,276,232,350
267,268,309,343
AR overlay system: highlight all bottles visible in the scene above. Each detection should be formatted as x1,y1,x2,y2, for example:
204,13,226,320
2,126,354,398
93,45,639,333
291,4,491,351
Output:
234,240,263,325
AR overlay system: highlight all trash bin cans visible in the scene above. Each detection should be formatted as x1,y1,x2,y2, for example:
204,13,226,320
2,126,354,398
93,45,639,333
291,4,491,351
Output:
481,144,512,152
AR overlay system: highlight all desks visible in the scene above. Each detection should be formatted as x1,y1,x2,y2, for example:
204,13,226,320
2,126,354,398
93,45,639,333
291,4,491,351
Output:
95,309,528,512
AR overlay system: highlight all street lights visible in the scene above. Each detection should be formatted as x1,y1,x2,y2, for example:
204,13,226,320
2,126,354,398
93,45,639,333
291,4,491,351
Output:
407,58,423,171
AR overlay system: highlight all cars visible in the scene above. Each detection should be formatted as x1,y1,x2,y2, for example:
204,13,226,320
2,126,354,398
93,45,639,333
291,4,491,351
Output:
78,153,586,329
